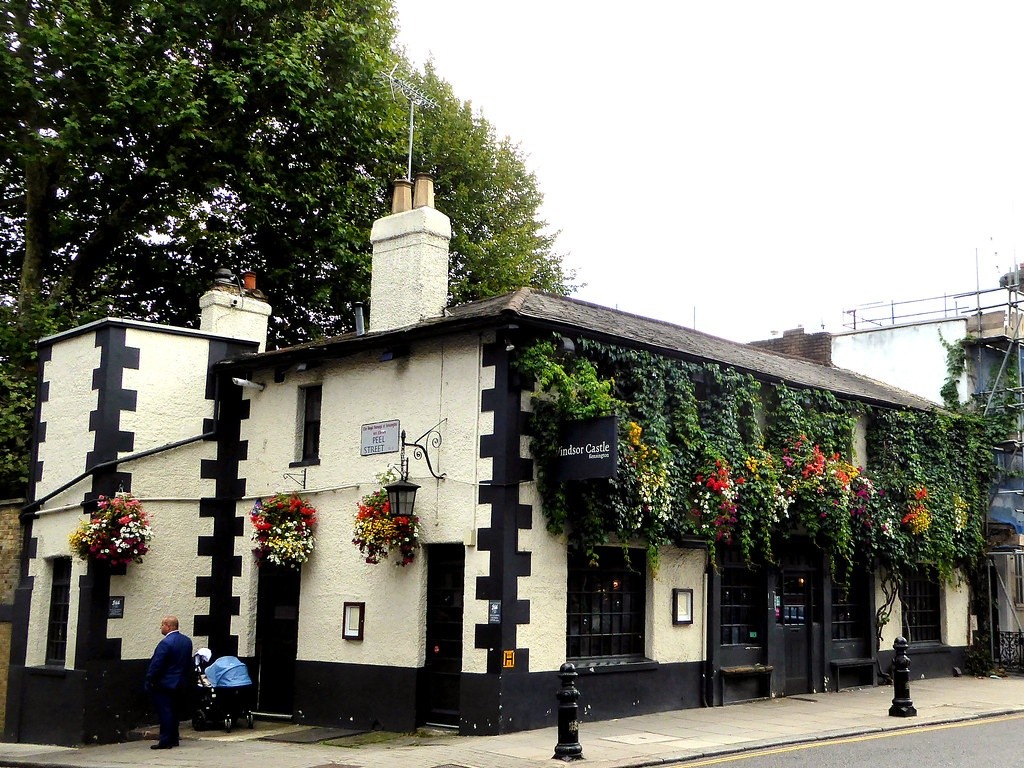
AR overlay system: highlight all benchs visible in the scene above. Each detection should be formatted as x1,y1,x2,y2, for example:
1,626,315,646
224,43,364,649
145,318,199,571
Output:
719,665,774,707
831,657,877,693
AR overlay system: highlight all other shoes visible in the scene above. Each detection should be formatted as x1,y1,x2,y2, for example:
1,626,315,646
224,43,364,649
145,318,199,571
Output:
150,742,180,749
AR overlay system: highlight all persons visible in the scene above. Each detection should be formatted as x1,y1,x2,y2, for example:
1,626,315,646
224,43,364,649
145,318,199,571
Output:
144,616,193,749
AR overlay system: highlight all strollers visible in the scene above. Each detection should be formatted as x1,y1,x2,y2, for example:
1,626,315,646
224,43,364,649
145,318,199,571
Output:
194,649,254,732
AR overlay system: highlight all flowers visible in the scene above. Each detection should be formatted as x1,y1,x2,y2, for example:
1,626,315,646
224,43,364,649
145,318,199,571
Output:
68,491,155,565
350,468,421,568
246,488,319,572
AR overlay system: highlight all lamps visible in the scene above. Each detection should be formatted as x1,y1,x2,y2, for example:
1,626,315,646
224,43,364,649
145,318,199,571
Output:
383,418,447,517
